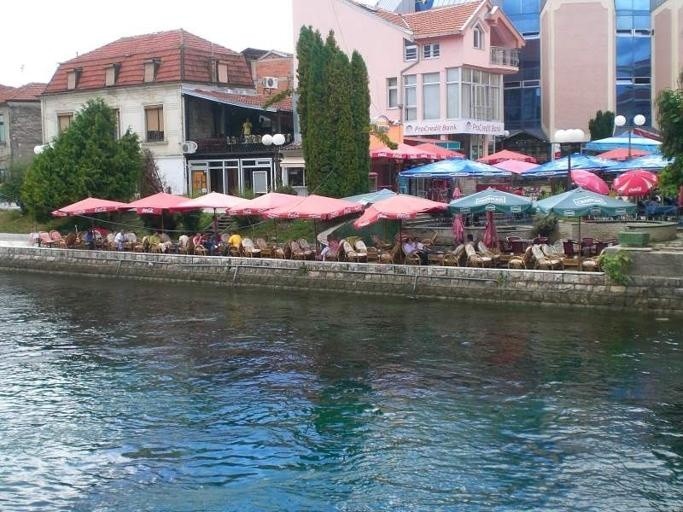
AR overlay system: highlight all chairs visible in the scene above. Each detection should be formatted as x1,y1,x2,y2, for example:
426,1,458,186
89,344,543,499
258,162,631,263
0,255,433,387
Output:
38,230,605,275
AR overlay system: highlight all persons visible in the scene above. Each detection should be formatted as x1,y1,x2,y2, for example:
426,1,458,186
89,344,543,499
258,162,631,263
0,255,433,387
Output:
81,225,476,267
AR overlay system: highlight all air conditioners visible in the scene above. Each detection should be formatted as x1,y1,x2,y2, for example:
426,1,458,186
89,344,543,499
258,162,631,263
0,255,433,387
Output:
262,76,278,90
180,142,195,155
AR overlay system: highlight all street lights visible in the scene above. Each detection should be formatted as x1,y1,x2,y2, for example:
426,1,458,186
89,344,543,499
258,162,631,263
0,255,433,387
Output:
550,128,590,190
261,133,286,189
614,114,647,157
